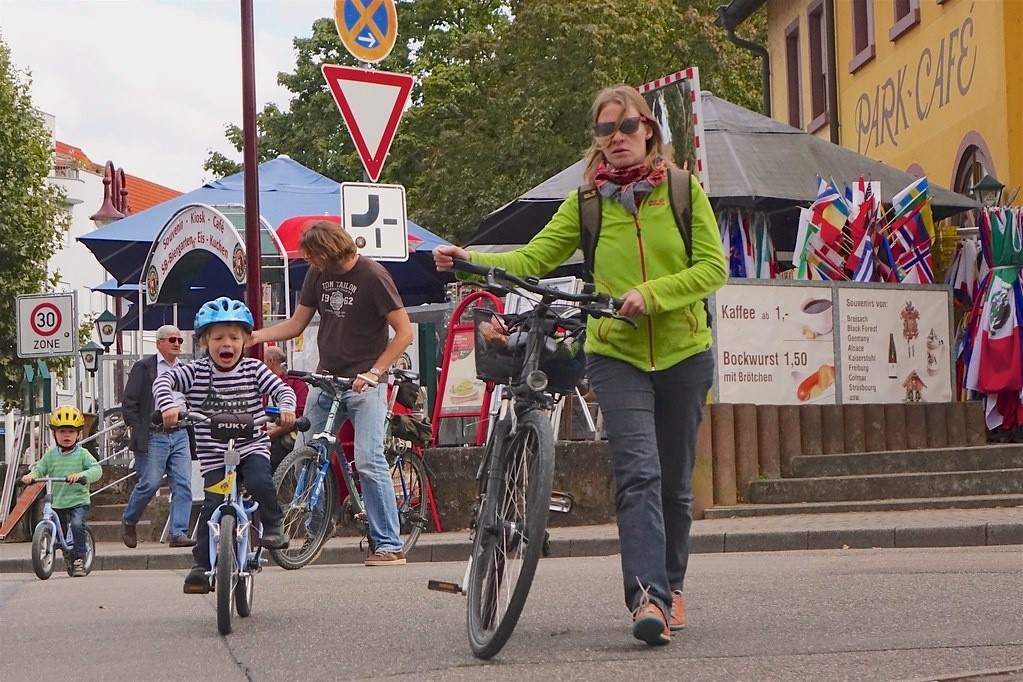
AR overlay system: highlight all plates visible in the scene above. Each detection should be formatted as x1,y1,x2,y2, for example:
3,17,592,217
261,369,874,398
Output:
450,388,477,398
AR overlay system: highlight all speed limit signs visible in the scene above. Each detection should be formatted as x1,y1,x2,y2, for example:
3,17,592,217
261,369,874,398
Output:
19,295,73,355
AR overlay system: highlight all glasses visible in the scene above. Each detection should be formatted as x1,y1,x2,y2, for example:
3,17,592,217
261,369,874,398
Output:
592,116,648,138
160,337,183,344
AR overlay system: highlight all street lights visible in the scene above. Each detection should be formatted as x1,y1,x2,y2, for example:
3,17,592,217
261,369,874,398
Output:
78,341,105,414
93,310,120,466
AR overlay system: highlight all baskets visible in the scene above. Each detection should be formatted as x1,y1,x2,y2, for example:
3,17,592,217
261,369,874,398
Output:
474,308,585,395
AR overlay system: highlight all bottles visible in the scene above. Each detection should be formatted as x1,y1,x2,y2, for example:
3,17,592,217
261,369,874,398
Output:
889,333,898,379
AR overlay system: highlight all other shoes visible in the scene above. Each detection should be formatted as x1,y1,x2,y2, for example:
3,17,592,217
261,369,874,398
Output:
169,535,197,547
121,517,136,548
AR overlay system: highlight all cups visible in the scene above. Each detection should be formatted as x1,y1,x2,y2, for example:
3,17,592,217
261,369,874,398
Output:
799,296,834,335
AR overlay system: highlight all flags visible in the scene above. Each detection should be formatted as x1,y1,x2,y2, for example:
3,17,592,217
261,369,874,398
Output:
713,206,780,279
943,206,1023,430
793,173,936,284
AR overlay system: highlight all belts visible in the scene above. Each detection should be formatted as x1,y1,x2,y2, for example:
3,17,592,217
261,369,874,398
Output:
149,426,180,432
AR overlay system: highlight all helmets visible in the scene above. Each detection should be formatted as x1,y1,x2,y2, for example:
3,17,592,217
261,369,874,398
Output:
194,296,253,338
48,405,85,431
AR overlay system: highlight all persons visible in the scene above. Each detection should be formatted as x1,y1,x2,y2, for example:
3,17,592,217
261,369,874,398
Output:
150,297,297,584
433,85,728,647
22,405,103,577
23,427,51,464
242,219,413,567
120,325,198,548
262,346,308,477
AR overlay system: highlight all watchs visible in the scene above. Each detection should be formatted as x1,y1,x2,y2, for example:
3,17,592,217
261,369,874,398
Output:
370,368,381,377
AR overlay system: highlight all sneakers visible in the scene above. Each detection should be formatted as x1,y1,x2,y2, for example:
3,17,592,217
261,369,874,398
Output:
261,521,285,548
630,576,672,646
670,590,686,630
185,562,209,584
72,558,87,576
364,550,406,566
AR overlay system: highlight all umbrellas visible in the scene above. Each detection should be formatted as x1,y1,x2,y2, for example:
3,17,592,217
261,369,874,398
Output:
455,90,986,266
76,153,460,332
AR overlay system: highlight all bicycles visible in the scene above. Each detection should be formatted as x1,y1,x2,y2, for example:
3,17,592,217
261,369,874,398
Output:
14,476,96,581
426,257,640,663
262,368,430,571
154,409,314,637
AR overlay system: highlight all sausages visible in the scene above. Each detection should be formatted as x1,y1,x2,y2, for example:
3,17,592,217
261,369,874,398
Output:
797,365,834,401
